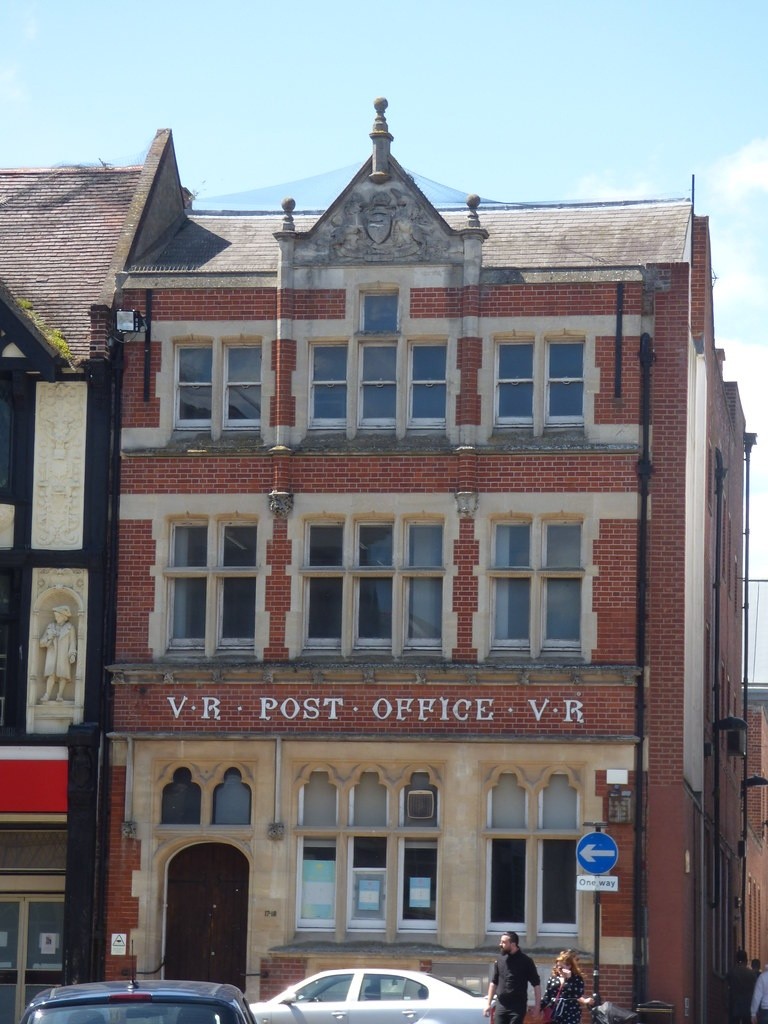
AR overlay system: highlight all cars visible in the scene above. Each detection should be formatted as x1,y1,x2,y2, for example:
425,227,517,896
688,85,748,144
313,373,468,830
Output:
215,968,497,1024
20,979,259,1024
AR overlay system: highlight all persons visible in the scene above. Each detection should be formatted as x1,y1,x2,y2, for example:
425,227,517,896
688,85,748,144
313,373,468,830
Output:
750,961,768,1024
483,930,541,1024
751,959,761,977
39,604,76,703
720,950,757,1024
533,950,595,1024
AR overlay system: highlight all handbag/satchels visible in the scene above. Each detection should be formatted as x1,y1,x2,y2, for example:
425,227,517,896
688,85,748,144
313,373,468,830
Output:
542,1007,554,1022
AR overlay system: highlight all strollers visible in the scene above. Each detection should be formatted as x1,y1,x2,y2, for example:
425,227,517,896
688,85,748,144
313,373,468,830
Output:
586,998,640,1024
